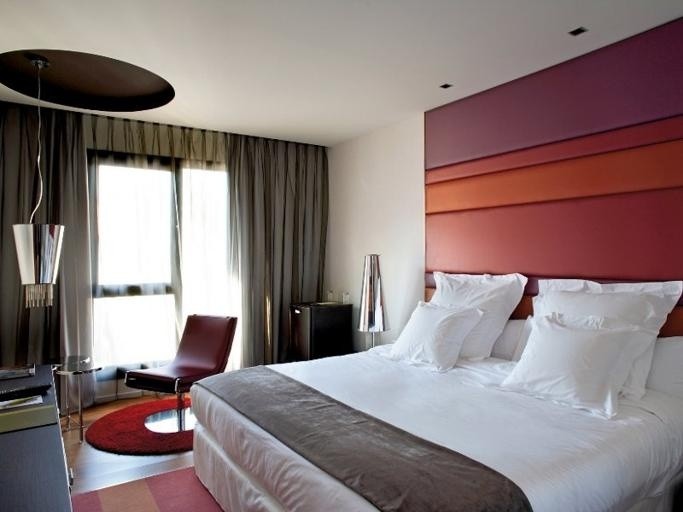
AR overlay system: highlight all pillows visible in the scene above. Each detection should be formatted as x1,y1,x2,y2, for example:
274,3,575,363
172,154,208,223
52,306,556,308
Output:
493,314,659,421
516,278,682,402
426,271,529,364
491,319,682,404
387,299,484,374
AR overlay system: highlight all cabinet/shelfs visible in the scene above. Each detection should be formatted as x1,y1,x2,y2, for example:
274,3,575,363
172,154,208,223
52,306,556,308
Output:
289,303,354,361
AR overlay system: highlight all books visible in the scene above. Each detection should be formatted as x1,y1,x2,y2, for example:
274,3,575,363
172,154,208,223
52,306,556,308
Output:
0,363,36,380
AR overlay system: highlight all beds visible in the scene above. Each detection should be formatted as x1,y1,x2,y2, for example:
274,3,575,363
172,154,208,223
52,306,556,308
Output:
189,344,683,512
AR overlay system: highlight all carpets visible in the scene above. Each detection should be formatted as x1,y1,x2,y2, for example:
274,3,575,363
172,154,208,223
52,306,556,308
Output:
83,397,193,456
72,467,224,511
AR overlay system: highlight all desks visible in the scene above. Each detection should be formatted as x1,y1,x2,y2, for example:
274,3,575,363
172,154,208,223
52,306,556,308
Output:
56,365,101,443
1,363,75,512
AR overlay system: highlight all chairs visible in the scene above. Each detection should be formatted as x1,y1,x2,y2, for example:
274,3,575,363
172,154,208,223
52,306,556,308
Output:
124,314,237,435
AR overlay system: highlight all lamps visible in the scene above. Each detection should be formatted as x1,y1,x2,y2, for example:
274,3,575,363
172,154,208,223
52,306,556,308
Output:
357,251,385,350
12,57,65,310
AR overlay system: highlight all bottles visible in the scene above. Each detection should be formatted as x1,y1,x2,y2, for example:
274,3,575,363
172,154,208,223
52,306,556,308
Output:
327,289,335,302
341,292,350,305
